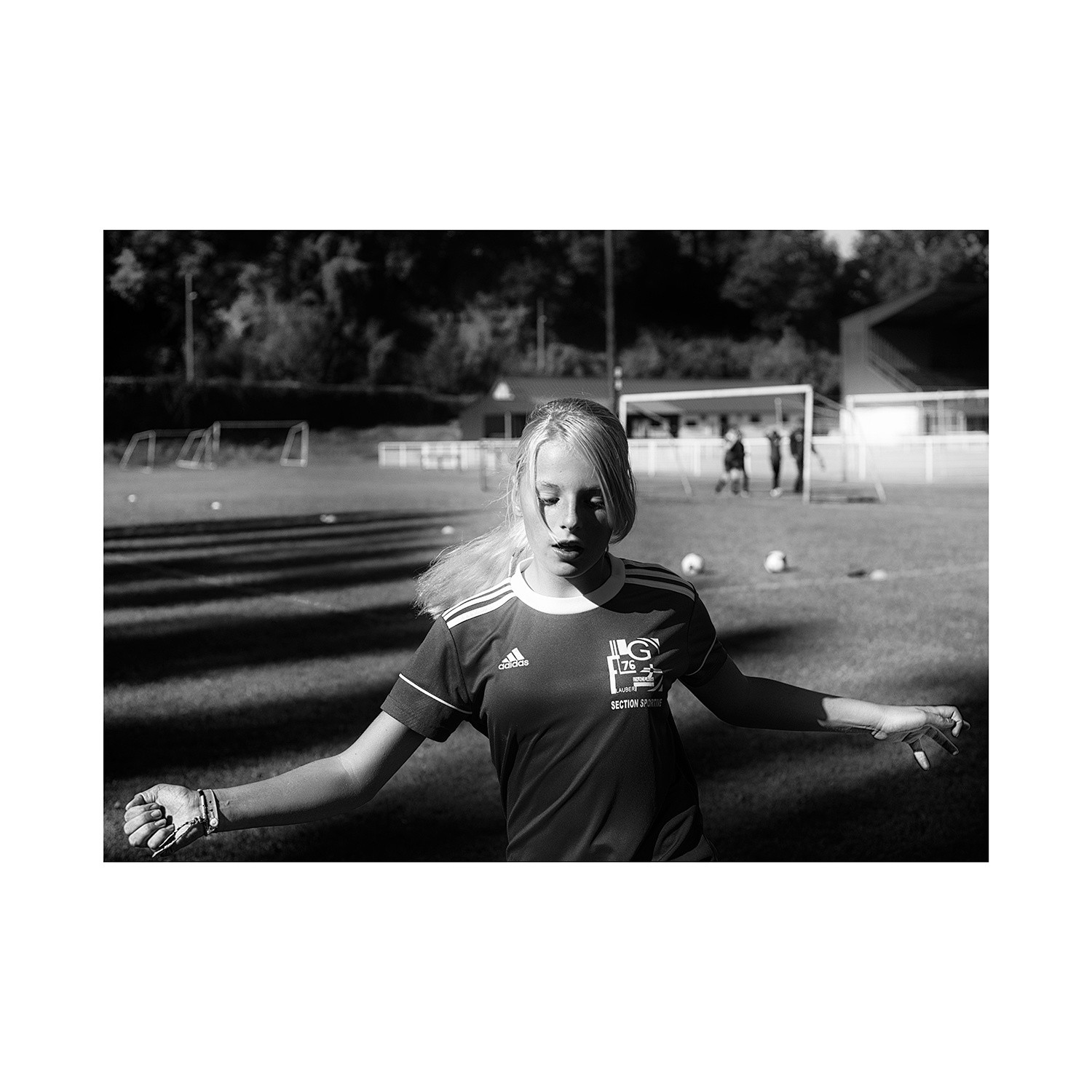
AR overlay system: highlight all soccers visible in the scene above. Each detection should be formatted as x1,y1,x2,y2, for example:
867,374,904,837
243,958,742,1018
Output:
763,550,787,576
679,552,707,578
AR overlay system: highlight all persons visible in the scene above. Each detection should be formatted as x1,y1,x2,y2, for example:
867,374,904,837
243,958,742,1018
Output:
766,430,782,497
712,428,751,497
789,419,817,493
122,399,971,863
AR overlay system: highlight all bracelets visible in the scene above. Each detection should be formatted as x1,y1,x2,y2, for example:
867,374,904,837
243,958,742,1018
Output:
152,788,219,858
